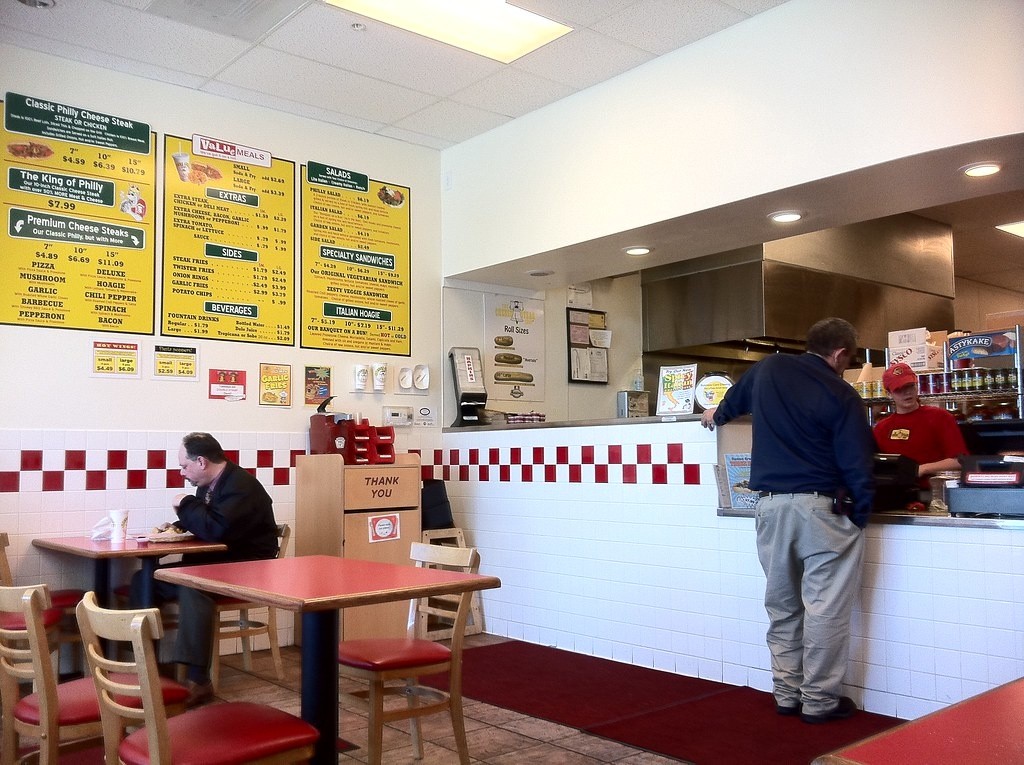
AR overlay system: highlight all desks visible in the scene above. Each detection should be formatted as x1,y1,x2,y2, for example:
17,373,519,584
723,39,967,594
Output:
151,551,500,765
31,530,231,663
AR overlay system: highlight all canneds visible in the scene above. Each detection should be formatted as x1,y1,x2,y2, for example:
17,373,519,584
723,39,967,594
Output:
852,367,1019,398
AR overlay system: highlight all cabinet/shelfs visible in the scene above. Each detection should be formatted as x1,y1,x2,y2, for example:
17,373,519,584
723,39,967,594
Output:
849,369,1020,429
293,450,430,653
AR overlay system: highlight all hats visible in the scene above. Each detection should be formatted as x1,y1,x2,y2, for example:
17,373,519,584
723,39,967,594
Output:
882,363,918,391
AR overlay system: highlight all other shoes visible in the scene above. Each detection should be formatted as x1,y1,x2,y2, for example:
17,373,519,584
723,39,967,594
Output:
802,696,857,724
773,696,801,716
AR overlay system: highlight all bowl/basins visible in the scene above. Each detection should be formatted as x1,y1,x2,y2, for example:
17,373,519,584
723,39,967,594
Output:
136,538,149,547
385,199,404,208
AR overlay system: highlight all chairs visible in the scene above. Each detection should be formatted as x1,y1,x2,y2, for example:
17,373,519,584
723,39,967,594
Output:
0,586,189,764
340,542,483,765
2,533,93,672
74,590,321,764
187,522,292,691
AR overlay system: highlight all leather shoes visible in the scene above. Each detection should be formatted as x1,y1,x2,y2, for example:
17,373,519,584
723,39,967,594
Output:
181,678,214,710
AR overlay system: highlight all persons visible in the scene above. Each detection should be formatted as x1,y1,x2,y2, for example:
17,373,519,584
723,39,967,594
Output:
129,427,283,708
703,316,886,727
871,363,970,504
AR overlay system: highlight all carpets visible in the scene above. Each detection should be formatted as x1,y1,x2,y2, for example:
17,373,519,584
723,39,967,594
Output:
406,636,740,728
576,686,911,765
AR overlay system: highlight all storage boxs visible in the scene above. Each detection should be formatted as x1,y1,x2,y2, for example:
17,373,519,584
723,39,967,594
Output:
886,328,949,369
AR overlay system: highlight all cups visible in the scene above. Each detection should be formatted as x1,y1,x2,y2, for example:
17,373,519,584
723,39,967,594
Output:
173,153,190,182
355,362,387,391
109,509,130,543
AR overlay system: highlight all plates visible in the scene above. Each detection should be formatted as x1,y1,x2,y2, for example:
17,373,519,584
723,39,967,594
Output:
399,364,430,390
134,533,195,542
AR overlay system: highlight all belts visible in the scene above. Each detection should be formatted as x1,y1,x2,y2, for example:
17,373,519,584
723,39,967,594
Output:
759,491,837,499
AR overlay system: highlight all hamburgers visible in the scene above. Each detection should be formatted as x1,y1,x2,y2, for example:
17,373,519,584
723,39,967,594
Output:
152,526,183,536
